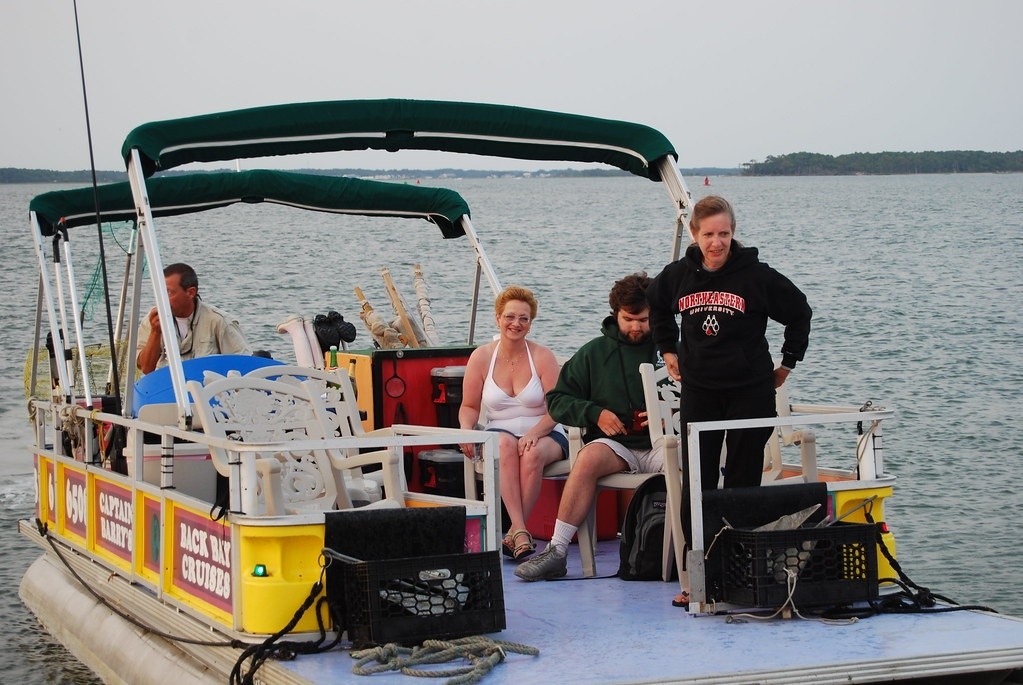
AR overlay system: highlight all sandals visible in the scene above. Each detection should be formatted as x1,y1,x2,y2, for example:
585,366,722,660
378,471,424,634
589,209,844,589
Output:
502,534,513,557
512,528,536,560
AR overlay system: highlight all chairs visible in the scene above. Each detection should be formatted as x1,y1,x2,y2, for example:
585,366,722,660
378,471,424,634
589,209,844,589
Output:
464,450,596,579
227,365,401,503
185,376,405,510
567,426,682,577
640,363,819,598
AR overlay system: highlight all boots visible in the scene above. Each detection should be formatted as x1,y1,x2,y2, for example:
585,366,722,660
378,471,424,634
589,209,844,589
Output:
328,311,357,343
276,313,315,368
316,315,341,358
302,315,326,370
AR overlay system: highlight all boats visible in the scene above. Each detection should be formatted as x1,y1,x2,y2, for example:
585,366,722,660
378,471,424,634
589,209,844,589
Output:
8,98,1023,685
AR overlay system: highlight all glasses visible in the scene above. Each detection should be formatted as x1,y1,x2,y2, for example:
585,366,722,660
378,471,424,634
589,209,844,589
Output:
500,313,531,325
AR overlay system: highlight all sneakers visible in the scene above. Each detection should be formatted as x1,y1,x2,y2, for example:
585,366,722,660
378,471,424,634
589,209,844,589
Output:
513,541,567,582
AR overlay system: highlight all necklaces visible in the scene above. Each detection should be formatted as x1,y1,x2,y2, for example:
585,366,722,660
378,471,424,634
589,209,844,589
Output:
498,348,526,372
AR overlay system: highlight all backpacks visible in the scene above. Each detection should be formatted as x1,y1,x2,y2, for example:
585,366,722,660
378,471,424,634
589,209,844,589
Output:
617,475,681,581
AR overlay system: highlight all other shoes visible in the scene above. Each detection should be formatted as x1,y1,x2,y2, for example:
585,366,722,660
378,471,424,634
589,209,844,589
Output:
672,591,689,607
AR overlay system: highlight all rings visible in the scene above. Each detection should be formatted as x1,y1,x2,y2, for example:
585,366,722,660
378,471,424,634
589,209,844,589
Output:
460,448,463,450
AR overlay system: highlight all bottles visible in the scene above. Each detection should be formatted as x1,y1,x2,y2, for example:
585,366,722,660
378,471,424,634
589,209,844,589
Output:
326,346,343,402
347,358,358,401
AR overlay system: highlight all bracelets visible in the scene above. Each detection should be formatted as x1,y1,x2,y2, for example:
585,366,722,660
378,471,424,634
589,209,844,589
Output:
781,366,790,370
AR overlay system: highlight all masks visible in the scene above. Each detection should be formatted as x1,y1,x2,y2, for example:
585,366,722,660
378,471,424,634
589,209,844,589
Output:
175,320,193,356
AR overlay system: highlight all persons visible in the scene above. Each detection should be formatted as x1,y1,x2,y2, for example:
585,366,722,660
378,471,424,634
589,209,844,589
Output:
515,274,682,581
136,263,254,375
458,286,569,560
648,197,814,608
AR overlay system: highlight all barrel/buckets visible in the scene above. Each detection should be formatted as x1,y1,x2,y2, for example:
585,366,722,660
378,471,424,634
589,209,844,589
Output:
431,365,468,452
420,449,465,502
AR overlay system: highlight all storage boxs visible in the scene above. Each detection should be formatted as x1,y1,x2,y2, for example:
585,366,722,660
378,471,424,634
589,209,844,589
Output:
342,549,506,644
719,519,880,608
